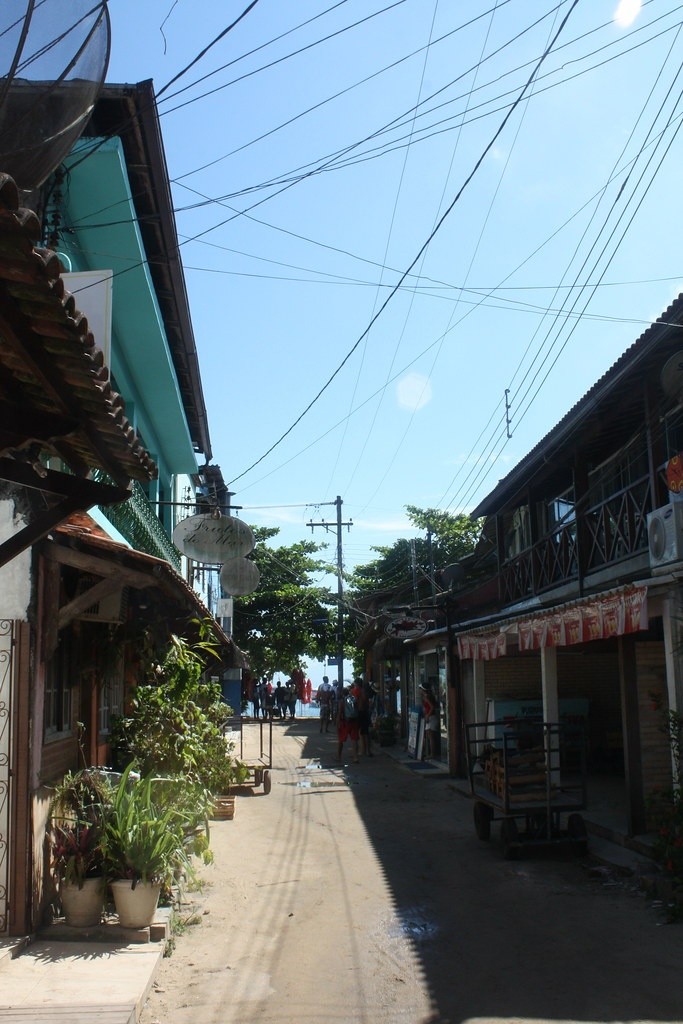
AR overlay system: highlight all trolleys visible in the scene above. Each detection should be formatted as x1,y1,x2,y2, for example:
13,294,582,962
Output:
216,715,274,794
463,716,586,846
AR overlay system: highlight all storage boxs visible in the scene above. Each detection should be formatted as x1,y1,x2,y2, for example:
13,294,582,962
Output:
208,796,237,821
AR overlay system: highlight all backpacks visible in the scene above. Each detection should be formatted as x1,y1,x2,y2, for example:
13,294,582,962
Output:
342,694,359,720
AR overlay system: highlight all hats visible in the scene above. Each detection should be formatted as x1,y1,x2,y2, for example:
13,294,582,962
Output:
418,684,428,690
332,680,338,683
323,686,328,691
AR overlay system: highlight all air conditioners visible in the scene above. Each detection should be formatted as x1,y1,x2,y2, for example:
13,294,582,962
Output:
72,574,129,625
646,501,683,569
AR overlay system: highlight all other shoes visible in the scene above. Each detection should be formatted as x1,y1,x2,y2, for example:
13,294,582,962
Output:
358,752,373,757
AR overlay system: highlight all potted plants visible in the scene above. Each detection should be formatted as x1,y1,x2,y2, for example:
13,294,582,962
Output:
101,757,200,930
43,816,108,927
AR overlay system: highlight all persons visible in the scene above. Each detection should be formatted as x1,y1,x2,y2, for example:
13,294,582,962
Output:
418,682,439,759
317,676,338,732
252,678,296,719
351,678,371,755
333,687,359,762
